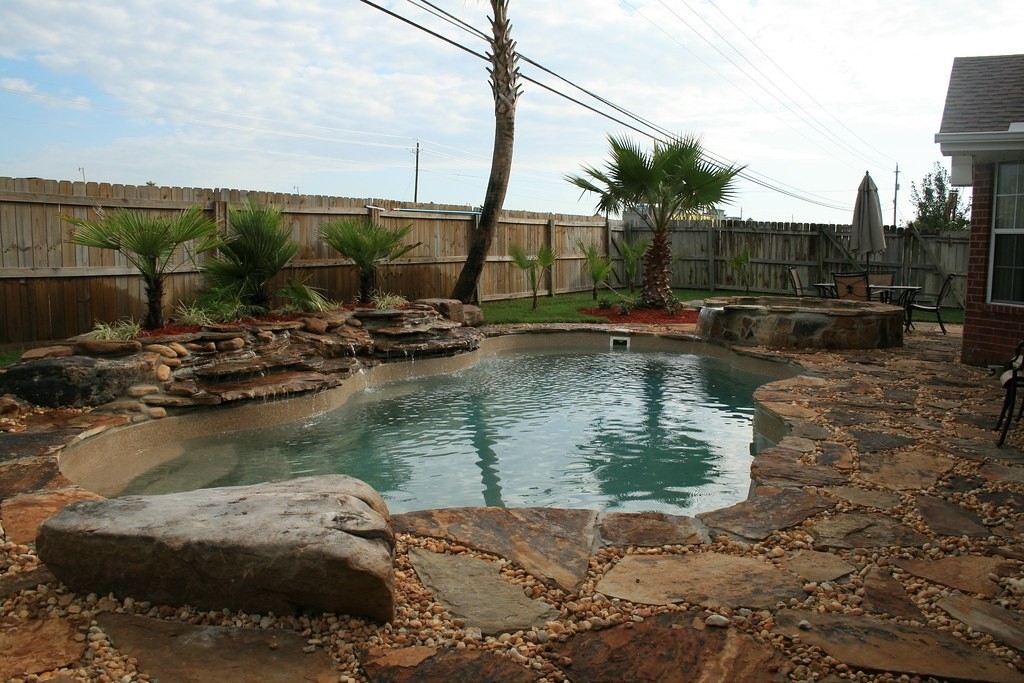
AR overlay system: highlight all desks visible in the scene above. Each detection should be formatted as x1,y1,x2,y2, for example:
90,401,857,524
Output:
813,283,922,333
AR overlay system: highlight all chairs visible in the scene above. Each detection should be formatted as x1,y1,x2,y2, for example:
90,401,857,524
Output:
864,270,896,305
904,274,955,335
788,267,834,299
830,271,871,302
994,337,1024,447
